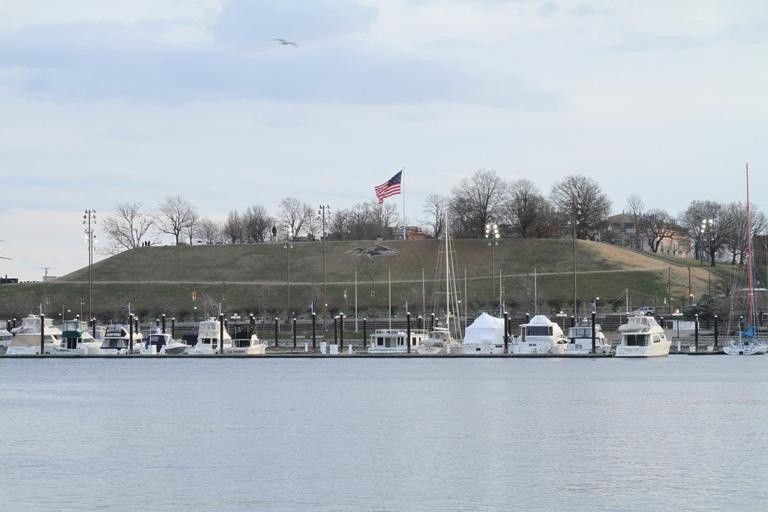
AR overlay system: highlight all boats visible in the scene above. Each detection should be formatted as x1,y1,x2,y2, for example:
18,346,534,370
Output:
367,212,613,355
0,302,265,354
614,309,672,358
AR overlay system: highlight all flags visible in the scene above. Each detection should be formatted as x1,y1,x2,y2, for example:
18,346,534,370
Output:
373,170,401,204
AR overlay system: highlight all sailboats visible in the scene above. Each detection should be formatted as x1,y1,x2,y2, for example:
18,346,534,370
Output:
723,160,768,355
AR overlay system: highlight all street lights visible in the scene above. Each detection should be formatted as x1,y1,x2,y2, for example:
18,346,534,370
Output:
282,230,294,316
316,202,331,336
82,208,97,321
